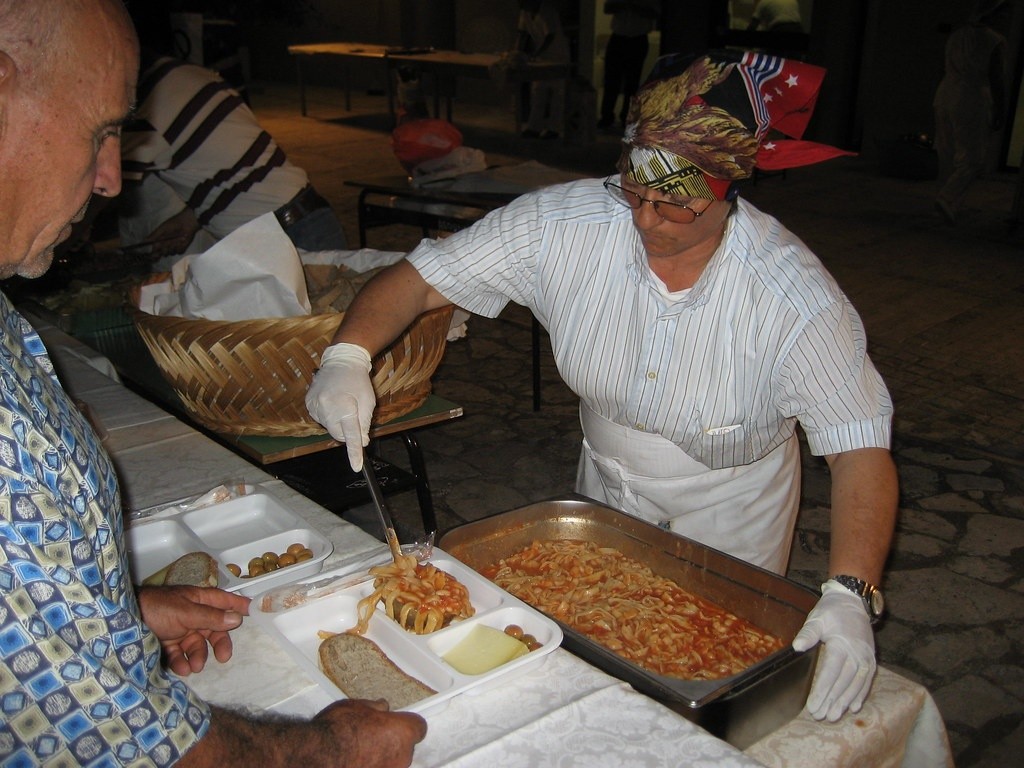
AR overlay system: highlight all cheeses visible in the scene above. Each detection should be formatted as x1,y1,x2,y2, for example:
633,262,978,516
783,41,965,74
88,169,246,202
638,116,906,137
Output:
441,622,531,676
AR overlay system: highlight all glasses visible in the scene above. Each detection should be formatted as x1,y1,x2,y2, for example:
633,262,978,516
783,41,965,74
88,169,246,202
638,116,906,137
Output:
604,167,715,225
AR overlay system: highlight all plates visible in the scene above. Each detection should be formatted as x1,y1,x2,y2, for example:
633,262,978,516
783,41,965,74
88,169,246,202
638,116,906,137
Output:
246,537,563,724
122,480,334,611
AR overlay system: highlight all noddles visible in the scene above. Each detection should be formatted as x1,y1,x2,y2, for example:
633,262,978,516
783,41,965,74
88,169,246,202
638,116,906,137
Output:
492,538,780,681
317,556,477,639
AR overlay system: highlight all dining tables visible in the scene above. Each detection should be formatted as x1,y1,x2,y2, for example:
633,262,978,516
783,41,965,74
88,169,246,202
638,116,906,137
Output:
12,303,773,767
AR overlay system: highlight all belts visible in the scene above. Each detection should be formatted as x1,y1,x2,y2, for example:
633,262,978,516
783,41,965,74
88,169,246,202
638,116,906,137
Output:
276,185,320,229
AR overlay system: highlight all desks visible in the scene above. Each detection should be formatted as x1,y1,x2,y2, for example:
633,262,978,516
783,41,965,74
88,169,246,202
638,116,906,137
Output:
342,163,593,414
385,52,568,154
740,667,952,768
149,377,463,547
288,41,435,114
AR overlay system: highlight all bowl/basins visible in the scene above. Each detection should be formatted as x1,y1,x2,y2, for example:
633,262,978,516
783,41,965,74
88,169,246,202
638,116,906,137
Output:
439,487,829,752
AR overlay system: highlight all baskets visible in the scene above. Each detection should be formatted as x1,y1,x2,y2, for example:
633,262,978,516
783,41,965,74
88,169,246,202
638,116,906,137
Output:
122,266,454,438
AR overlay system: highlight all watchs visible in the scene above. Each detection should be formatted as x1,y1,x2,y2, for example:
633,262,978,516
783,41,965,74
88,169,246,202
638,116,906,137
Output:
827,575,884,624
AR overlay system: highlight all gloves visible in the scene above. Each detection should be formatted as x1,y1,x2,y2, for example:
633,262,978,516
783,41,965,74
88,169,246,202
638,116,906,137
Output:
304,343,376,473
792,578,878,723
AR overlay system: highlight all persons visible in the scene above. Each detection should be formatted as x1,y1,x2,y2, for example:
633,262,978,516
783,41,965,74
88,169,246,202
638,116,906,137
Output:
933,1,1007,225
0,0,427,767
307,49,898,723
68,61,350,255
511,0,567,139
746,0,806,62
596,0,660,131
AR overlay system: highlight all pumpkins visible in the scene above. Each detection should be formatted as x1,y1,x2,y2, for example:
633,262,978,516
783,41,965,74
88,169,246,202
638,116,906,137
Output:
392,118,462,174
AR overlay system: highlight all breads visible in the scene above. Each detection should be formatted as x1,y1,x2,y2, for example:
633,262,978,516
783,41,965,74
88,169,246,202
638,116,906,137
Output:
317,633,438,710
302,261,391,316
142,551,218,588
